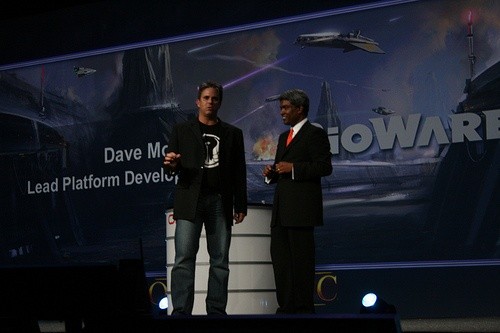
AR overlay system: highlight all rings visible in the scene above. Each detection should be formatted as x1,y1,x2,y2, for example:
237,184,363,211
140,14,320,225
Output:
278,168,281,171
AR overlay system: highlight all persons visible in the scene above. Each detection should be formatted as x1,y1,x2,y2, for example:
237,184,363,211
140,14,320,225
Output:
163,79,249,319
262,89,334,315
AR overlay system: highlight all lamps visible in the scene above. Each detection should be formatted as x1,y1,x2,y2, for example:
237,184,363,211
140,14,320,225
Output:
359,292,397,315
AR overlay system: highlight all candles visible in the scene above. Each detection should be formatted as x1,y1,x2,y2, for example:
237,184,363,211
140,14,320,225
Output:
467,8,473,35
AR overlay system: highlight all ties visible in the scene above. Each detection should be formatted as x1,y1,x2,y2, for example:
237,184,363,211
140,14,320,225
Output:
286,128,294,147
